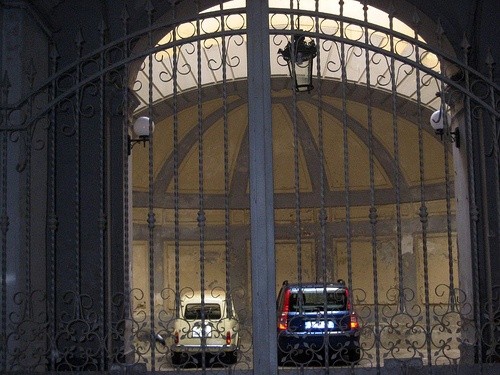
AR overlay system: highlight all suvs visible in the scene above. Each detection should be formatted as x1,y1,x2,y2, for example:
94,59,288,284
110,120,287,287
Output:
276,279,362,364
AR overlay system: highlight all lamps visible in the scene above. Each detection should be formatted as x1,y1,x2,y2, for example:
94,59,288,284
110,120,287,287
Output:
127,116,155,156
429,110,460,148
275,29,319,95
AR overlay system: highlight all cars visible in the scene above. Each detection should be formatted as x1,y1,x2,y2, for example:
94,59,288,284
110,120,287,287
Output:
170,289,240,364
